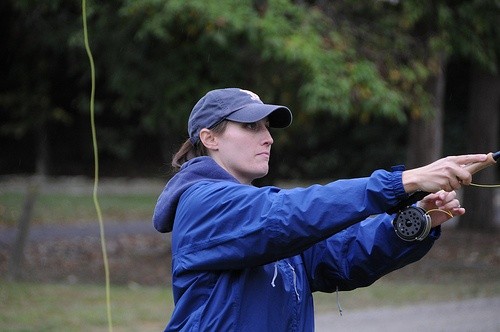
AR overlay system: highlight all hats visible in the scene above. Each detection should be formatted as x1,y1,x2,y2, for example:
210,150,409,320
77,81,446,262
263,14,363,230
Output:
188,88,292,145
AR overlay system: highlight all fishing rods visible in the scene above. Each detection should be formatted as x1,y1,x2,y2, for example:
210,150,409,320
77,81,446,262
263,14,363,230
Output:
258,149,497,268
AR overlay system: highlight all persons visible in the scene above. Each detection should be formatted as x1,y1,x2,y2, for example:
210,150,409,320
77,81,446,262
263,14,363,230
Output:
153,87,487,332
